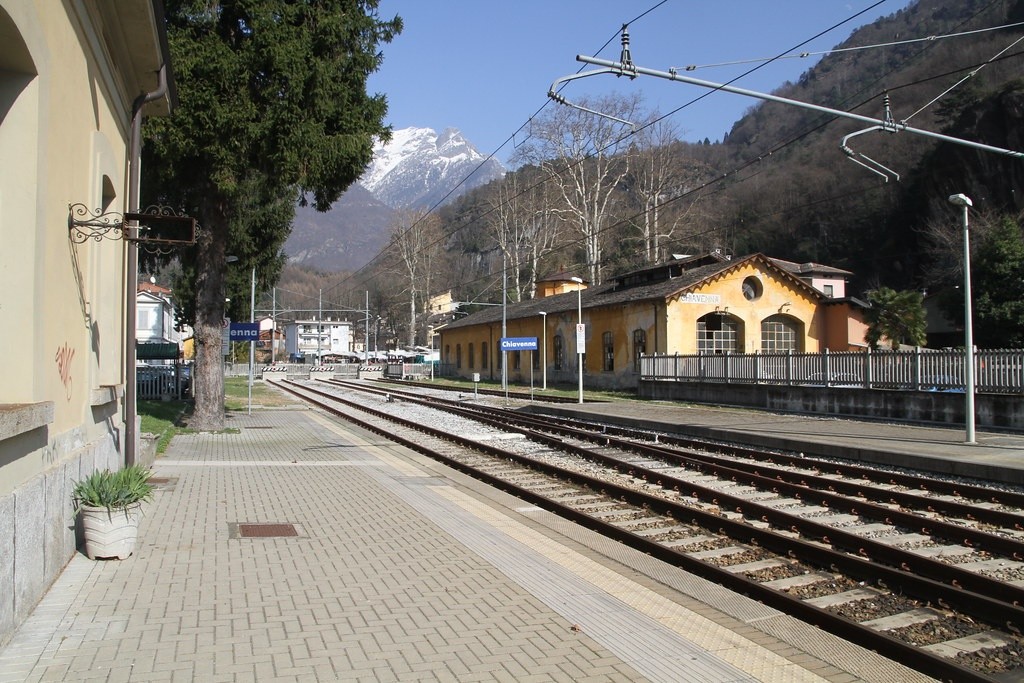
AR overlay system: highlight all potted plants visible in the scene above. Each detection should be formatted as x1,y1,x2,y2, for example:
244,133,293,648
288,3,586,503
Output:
67,463,160,561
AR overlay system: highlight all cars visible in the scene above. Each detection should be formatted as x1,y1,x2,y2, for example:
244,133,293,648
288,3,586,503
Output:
900,373,967,395
799,371,873,398
317,346,439,371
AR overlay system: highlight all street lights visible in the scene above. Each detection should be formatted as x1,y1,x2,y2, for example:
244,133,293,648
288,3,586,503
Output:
539,311,550,391
426,324,436,381
569,275,587,407
948,193,984,445
